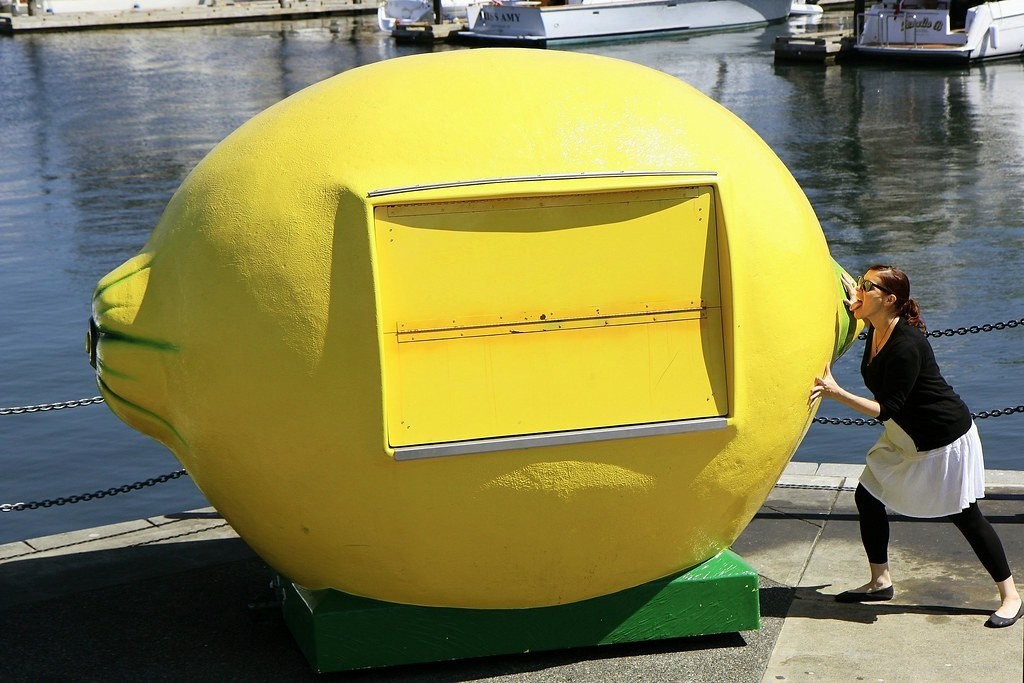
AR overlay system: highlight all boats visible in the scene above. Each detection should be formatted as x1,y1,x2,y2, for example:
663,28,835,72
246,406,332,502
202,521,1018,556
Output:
445,0,805,51
379,1,502,42
851,0,1024,67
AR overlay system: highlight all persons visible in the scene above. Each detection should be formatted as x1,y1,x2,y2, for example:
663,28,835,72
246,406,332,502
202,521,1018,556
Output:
809,264,1024,629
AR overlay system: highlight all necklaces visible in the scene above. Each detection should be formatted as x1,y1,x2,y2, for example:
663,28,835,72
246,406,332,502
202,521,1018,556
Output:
875,314,897,354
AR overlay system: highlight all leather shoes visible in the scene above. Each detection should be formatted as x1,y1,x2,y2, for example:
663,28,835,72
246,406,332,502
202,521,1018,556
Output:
834,583,894,602
987,601,1024,627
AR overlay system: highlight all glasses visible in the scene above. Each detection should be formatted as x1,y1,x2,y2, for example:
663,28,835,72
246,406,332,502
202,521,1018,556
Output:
857,275,892,296
